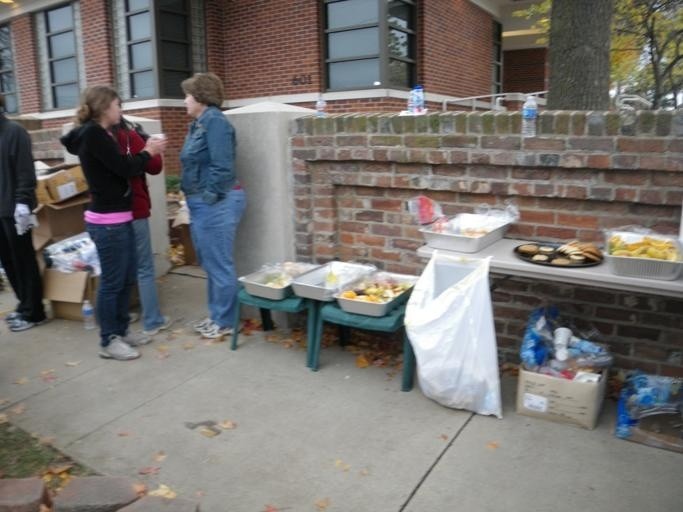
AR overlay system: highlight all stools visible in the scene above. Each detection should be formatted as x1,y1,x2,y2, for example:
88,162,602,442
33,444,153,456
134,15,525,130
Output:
230,288,416,392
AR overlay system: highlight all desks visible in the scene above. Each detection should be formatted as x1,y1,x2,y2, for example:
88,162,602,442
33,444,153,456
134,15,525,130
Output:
413,237,683,298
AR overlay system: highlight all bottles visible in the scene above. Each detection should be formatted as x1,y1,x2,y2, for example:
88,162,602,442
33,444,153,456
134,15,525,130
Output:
406,90,414,114
315,93,328,117
554,334,607,361
413,85,425,114
520,94,538,138
80,299,97,330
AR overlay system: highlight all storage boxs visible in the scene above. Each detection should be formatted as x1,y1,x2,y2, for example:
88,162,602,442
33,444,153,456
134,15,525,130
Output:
515,360,608,429
29,162,139,323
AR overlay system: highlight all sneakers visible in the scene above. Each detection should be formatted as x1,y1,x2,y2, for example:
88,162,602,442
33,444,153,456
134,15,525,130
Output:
194,316,227,332
98,334,141,361
142,315,175,335
8,314,48,331
200,320,241,339
115,326,152,346
4,305,35,322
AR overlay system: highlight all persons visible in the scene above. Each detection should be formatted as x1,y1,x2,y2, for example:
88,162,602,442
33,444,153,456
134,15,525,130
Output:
178,71,247,340
59,84,169,359
108,116,172,337
0,94,49,333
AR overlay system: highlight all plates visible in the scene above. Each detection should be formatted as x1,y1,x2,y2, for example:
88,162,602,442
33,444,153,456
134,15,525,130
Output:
514,243,604,268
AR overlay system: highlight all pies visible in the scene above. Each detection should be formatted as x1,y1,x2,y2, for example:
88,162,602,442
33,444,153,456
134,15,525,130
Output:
518,240,603,266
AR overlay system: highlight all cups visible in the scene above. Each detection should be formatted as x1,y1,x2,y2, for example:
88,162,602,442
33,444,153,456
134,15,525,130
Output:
553,325,572,350
149,132,164,140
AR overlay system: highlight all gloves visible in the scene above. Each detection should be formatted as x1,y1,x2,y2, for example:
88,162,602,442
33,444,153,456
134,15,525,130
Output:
14,203,31,227
